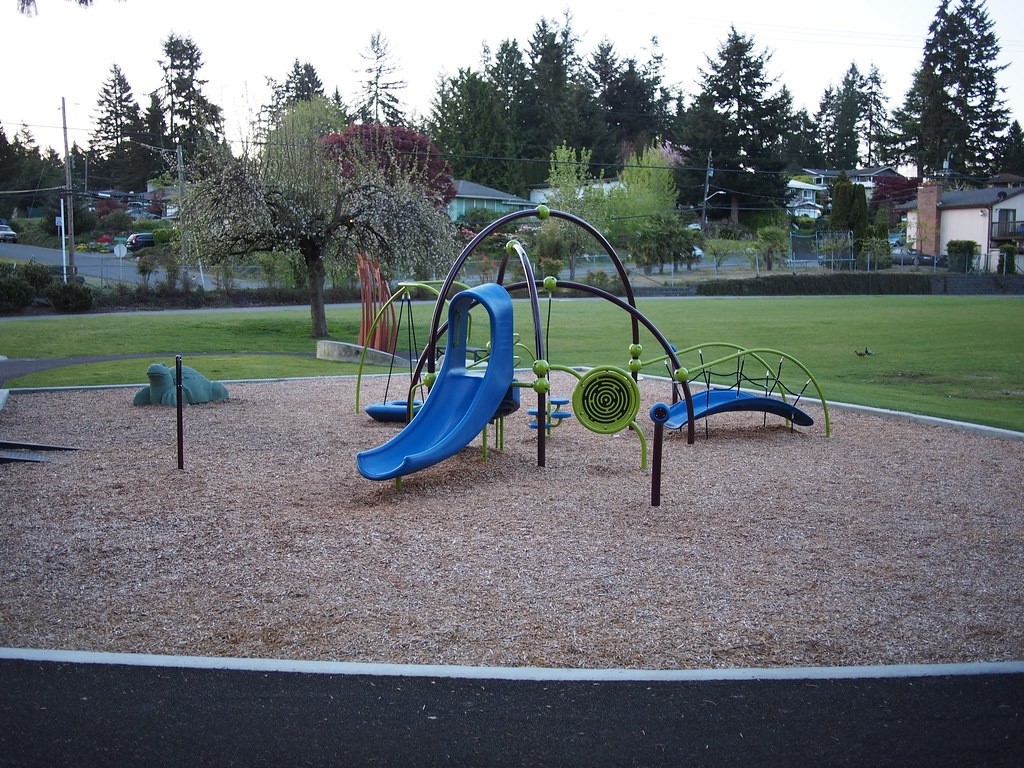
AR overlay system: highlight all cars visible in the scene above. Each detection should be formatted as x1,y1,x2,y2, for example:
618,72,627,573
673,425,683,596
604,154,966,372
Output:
679,245,704,261
689,223,701,231
81,204,100,212
124,233,170,253
888,247,938,266
0,224,17,243
125,209,161,221
125,201,152,208
888,233,907,247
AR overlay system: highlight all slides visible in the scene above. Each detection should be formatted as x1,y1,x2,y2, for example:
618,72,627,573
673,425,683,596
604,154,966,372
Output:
355,282,516,482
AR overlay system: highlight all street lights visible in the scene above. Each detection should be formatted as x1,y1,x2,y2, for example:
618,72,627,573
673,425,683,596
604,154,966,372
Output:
700,190,727,230
